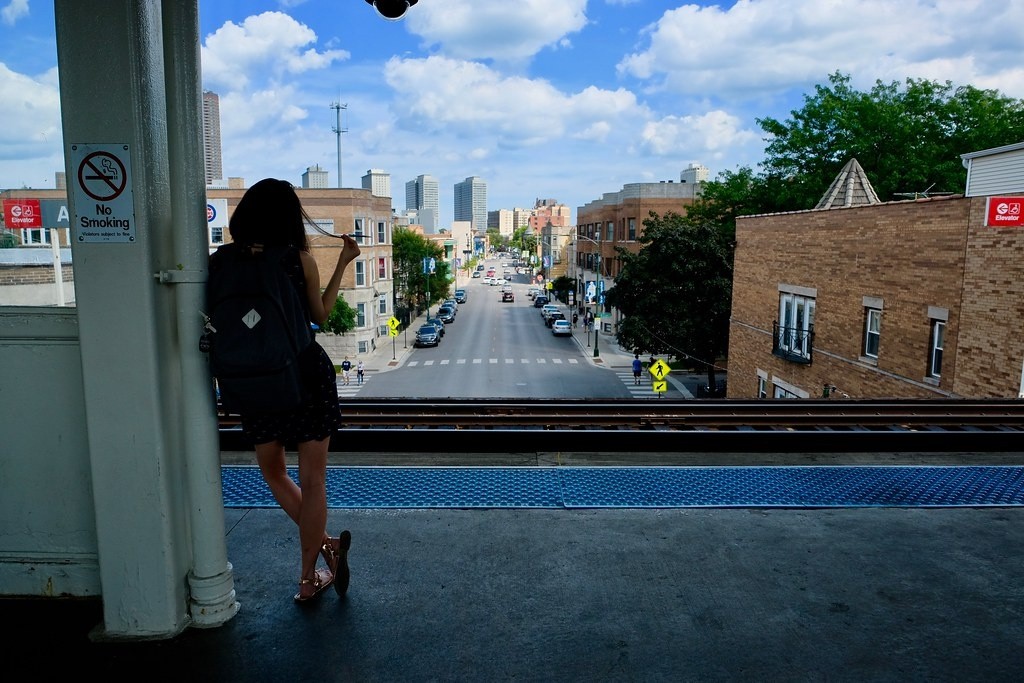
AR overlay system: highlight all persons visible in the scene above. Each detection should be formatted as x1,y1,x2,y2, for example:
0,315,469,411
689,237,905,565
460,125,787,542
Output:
632,354,642,384
573,311,579,329
646,357,657,380
583,312,595,334
357,361,365,386
340,356,352,386
199,178,361,608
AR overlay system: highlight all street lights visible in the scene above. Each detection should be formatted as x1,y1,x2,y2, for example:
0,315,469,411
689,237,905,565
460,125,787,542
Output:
538,240,552,301
568,233,601,357
528,242,535,276
517,241,527,266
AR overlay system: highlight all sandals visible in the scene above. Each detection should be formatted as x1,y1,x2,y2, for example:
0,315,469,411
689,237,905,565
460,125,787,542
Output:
293,570,334,605
322,529,351,597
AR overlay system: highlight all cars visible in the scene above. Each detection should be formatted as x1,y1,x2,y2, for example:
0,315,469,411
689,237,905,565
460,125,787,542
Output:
454,289,467,302
473,272,480,278
436,299,458,323
483,262,511,285
512,254,519,259
415,319,446,347
501,285,515,302
477,265,484,270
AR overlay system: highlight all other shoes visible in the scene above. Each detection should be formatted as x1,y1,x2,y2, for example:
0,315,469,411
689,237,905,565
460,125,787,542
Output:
638,380,640,384
344,383,346,385
358,384,360,386
346,383,349,385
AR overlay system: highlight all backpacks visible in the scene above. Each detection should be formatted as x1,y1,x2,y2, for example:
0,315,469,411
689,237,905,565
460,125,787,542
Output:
209,248,315,378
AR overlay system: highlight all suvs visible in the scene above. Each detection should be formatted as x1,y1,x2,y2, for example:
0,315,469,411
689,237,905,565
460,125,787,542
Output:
541,305,571,336
528,285,549,308
